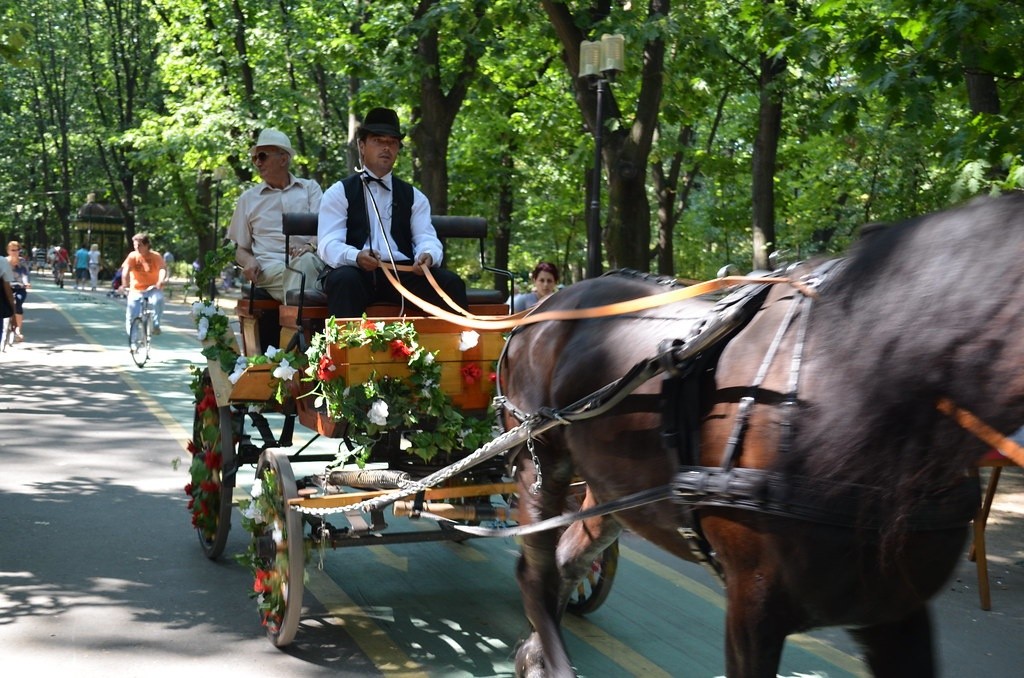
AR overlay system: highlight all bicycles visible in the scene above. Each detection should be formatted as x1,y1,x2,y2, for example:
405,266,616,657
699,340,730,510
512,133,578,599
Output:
113,284,160,368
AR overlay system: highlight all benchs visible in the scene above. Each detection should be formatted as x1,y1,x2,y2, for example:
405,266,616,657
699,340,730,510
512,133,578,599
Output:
279,212,514,352
235,243,283,357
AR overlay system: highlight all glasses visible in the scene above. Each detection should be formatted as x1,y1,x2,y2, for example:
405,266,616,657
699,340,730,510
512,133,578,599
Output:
10,248,20,251
251,152,285,164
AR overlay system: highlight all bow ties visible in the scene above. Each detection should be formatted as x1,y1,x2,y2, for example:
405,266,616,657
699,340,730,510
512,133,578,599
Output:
362,170,391,191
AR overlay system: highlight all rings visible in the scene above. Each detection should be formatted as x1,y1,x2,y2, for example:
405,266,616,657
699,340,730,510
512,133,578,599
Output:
299,248,302,251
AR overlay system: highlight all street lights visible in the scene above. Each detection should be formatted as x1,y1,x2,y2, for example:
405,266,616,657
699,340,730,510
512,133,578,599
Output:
577,32,626,279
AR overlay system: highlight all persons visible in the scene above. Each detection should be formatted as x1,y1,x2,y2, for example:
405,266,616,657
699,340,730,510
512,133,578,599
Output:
163,252,173,277
118,234,166,352
316,107,468,316
227,128,323,360
509,262,558,314
73,244,103,291
0,241,30,342
32,243,70,288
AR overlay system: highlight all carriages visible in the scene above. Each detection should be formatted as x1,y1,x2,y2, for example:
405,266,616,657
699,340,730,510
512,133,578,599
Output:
190,185,1024,678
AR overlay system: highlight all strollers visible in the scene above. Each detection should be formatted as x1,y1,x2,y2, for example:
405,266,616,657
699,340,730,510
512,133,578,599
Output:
107,270,125,299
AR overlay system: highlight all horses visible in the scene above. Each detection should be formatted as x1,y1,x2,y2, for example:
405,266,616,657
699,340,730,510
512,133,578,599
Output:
501,193,1024,678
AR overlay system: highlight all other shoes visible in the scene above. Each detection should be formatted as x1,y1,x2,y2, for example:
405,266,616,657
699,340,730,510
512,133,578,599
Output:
56,280,84,290
153,326,160,335
16,330,25,342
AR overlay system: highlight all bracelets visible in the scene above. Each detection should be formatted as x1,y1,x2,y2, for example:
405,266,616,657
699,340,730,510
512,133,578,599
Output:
305,242,317,253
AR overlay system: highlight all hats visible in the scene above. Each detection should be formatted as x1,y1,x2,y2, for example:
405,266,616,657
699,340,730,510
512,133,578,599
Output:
250,129,296,158
358,107,406,141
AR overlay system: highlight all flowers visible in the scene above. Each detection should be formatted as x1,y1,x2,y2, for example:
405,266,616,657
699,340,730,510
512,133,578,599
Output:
184,301,511,632
